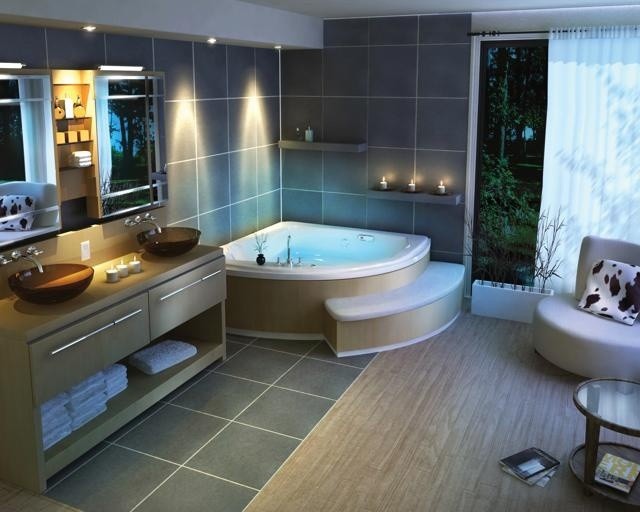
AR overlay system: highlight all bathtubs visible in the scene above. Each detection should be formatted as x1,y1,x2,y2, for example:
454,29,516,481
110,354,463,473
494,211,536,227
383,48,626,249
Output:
220,218,432,282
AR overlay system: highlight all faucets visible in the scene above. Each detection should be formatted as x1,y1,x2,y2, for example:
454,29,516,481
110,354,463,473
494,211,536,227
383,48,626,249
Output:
285,234,292,264
122,212,163,234
0,245,46,275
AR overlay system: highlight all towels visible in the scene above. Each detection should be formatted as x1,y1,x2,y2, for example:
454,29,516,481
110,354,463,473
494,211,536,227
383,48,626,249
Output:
36,335,198,449
68,151,93,170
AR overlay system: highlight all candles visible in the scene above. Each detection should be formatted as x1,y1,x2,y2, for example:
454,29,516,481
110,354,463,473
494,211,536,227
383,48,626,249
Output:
436,179,445,194
378,175,387,190
106,255,140,285
406,177,416,192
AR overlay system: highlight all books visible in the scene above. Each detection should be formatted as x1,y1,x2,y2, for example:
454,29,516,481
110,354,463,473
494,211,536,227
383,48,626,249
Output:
499,447,560,488
596,453,640,492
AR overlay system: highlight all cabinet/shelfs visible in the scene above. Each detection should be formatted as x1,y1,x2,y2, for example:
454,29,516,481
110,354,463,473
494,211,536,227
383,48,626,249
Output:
0,253,228,496
55,117,95,171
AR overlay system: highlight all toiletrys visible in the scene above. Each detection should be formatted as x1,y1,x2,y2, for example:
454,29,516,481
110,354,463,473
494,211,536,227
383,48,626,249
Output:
74,97,85,118
55,97,65,119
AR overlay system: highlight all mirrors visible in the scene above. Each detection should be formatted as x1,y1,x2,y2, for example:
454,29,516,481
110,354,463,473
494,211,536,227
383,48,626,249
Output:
95,73,168,220
0,74,63,246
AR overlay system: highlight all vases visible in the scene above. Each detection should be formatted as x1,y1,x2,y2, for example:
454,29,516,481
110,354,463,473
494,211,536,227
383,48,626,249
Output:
256,254,266,265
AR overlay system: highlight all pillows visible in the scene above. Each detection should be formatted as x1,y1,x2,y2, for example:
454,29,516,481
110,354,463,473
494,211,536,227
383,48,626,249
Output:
578,256,640,326
0,195,35,231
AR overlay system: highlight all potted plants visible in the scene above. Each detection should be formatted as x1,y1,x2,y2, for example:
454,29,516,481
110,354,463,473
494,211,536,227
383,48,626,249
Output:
470,144,565,325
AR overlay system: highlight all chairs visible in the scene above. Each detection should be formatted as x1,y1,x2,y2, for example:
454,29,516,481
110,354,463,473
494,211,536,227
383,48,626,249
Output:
530,233,639,381
0,178,59,244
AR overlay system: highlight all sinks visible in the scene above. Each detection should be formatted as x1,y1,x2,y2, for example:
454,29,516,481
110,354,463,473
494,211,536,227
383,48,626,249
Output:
137,225,202,256
8,263,94,302
